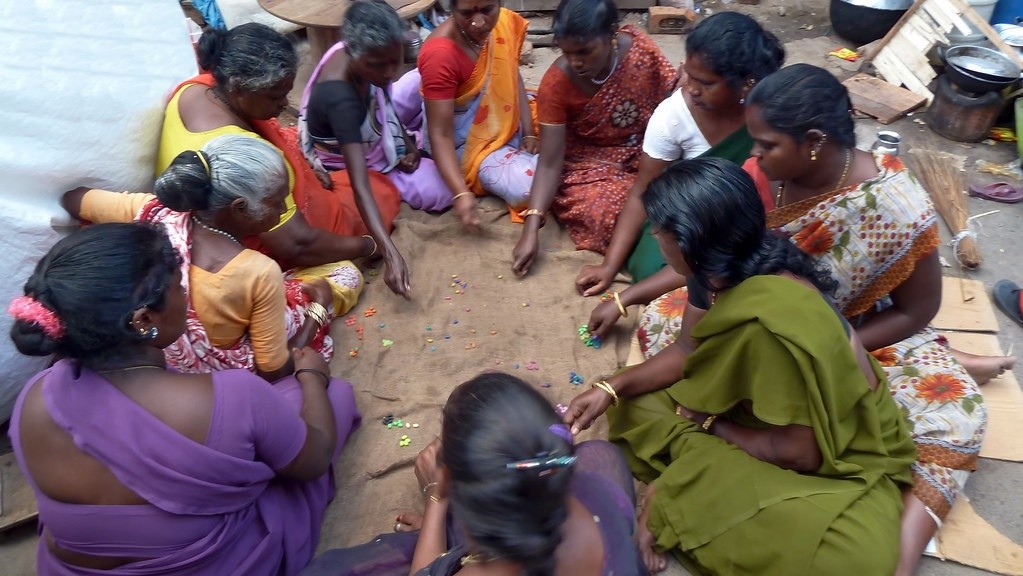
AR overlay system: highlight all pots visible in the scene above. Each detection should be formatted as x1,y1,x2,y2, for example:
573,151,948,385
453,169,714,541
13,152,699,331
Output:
937,45,1023,92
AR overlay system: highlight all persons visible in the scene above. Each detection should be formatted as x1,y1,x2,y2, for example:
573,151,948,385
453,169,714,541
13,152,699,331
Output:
7,222,363,576
563,157,918,576
574,12,1017,385
512,0,679,277
588,63,988,576
417,0,540,232
63,134,364,383
154,23,401,269
298,0,453,301
304,372,648,576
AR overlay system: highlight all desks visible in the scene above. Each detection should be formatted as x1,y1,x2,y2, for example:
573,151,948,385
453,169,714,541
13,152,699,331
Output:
256,0,437,68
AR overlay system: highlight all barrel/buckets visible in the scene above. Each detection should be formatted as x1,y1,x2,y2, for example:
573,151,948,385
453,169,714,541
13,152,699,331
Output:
937,0,1023,49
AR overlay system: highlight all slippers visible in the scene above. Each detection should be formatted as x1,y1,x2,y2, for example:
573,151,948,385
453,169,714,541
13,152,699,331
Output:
967,182,1023,202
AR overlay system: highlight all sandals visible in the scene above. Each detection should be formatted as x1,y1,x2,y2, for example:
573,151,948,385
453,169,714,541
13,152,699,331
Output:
993,279,1023,324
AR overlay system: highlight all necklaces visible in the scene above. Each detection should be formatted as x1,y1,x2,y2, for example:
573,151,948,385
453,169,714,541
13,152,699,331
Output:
97,366,163,373
460,553,500,565
212,89,229,106
460,28,485,48
192,216,239,244
775,149,850,209
712,291,716,304
590,56,618,84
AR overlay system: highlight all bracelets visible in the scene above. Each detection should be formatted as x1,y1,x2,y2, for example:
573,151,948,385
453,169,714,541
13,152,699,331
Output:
424,482,438,492
702,415,716,429
304,302,328,327
522,136,539,141
524,209,547,228
614,292,628,318
452,192,474,201
592,381,619,408
362,235,377,257
295,369,330,389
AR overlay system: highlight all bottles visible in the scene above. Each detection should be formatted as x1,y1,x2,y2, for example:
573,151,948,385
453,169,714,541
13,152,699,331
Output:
871,130,903,162
